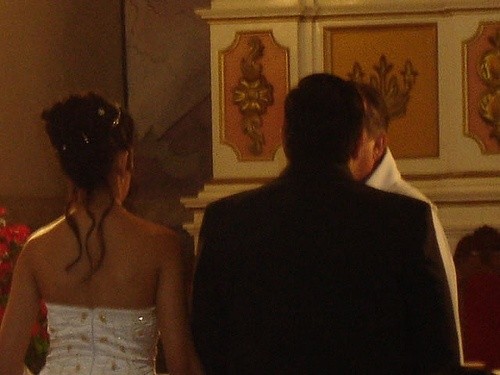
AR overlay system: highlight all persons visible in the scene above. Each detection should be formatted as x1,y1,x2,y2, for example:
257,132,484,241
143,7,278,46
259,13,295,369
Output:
193,73,464,375
0,93,204,375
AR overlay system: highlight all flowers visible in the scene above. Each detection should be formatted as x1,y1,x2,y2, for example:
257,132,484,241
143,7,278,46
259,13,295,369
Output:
0,207,50,358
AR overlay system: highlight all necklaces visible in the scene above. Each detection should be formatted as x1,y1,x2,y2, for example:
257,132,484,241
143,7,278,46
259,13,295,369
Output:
97,201,124,208
